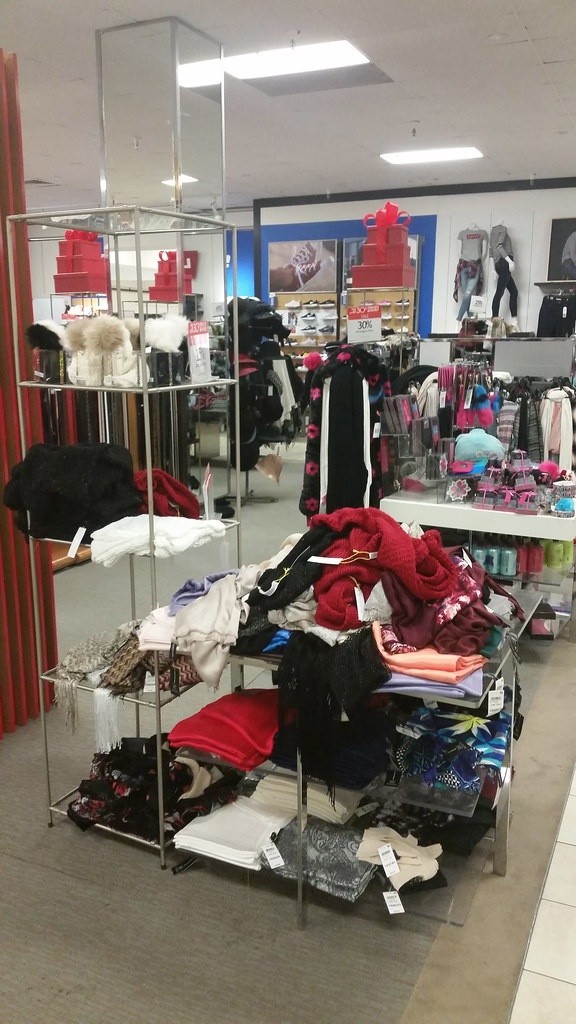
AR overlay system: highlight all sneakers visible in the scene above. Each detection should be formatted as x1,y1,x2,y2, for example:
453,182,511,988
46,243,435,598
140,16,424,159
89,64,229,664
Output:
280,297,411,372
283,256,334,292
290,241,322,268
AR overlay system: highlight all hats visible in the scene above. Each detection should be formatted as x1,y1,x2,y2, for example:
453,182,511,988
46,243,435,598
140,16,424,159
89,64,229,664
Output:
224,294,295,472
32,303,193,387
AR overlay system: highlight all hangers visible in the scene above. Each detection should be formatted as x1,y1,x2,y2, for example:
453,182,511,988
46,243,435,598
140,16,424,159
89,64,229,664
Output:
484,366,576,401
378,345,393,365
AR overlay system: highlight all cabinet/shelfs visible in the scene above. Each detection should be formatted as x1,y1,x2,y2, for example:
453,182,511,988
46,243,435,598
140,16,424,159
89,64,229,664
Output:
269,294,338,356
379,487,576,644
5,205,242,870
339,284,416,341
174,591,576,932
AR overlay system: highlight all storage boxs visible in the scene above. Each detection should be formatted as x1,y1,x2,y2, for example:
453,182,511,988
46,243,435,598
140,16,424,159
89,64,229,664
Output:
53,239,110,293
382,394,576,518
528,604,556,641
351,224,417,288
148,260,192,300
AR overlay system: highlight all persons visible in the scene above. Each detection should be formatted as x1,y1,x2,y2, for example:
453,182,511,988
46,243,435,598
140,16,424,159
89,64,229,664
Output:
488,220,521,332
270,240,335,292
453,222,489,332
